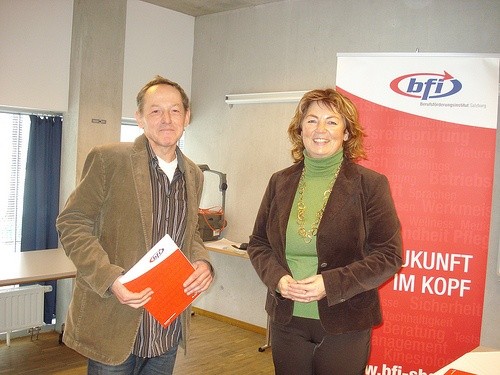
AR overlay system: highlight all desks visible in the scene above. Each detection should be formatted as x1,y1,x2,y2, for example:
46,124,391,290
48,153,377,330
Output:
0,248,77,288
191,240,271,352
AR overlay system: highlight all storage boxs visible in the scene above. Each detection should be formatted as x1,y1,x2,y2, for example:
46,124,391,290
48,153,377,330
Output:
198,210,222,242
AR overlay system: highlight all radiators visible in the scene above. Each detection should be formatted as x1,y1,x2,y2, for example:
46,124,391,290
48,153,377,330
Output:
0,284,52,334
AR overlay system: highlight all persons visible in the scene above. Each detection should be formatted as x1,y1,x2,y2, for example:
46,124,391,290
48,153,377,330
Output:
247,88,402,375
56,75,215,375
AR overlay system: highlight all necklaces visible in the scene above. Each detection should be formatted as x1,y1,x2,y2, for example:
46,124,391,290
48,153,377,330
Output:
296,162,341,244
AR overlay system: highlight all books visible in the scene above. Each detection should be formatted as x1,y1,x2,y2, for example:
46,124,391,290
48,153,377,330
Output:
119,234,205,329
